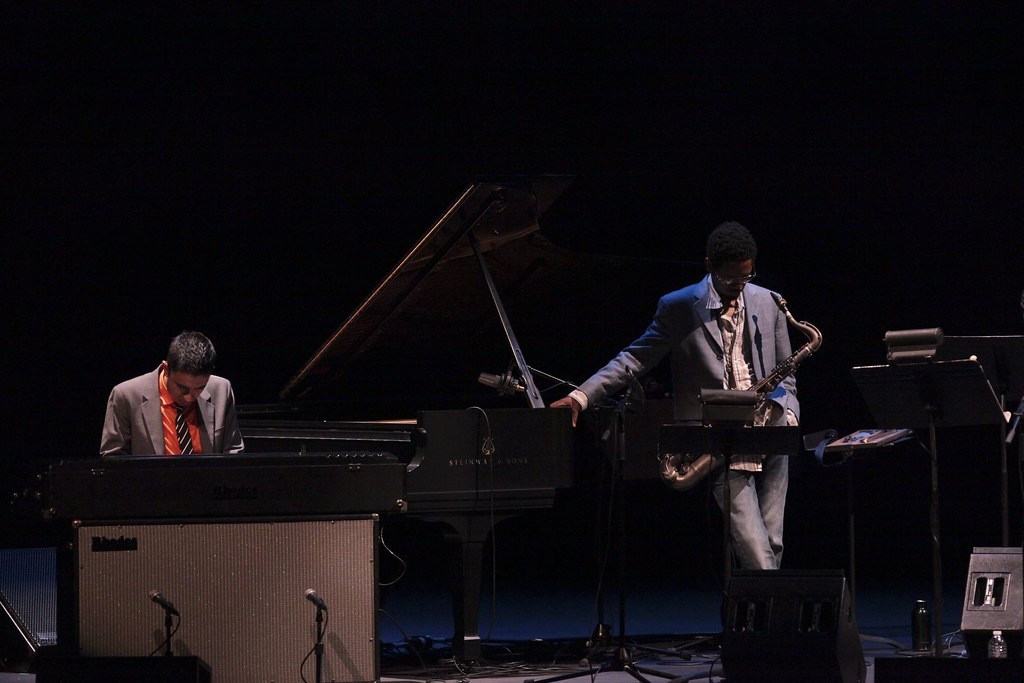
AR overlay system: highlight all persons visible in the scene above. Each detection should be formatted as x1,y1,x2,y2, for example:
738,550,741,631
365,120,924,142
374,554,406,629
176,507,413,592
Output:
99,330,246,457
546,219,801,572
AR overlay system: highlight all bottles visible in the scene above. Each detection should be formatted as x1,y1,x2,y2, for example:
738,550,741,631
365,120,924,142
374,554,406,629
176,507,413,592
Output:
987,631,1006,658
912,599,931,651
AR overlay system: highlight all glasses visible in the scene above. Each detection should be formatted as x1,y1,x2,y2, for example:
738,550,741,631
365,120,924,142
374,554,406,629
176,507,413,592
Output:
713,263,756,286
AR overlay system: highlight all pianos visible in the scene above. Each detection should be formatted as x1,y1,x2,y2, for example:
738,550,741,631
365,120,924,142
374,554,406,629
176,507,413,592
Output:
14,171,584,668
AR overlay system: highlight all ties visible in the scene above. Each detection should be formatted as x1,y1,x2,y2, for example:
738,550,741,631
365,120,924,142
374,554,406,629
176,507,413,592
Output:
174,403,194,454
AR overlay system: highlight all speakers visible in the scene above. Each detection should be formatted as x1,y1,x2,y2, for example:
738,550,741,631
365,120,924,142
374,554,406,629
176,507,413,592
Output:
960,547,1024,660
36,654,212,683
73,514,381,683
720,568,867,683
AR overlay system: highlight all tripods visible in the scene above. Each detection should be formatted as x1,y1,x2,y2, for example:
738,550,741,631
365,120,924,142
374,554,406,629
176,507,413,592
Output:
524,392,693,683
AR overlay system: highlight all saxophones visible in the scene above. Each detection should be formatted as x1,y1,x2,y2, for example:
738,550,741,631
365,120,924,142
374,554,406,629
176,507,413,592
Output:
658,290,825,493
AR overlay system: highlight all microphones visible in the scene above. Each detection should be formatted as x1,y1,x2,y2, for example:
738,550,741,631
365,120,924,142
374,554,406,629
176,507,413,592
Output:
304,589,327,611
625,366,647,401
478,372,525,395
149,590,179,616
